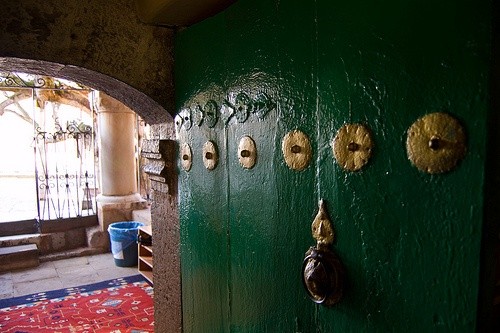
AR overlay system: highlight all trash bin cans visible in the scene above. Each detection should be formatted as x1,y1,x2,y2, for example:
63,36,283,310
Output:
107,221,143,267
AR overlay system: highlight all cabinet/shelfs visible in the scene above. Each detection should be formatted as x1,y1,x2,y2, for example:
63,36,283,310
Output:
137,226,153,286
